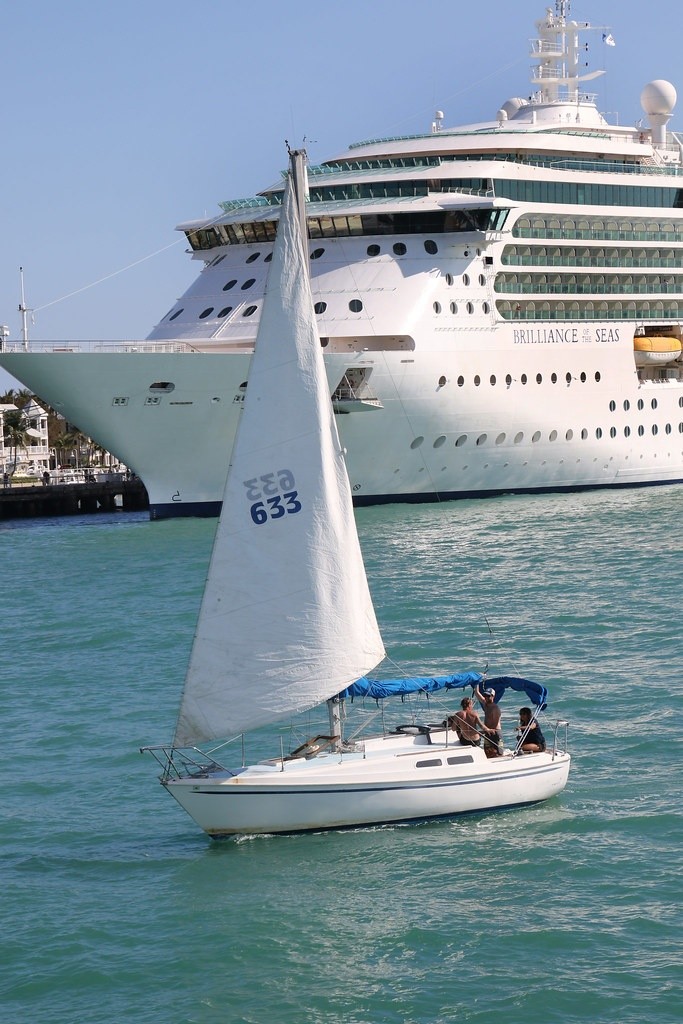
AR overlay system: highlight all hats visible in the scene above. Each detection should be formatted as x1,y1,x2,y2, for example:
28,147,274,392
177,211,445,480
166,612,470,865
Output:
484,688,495,696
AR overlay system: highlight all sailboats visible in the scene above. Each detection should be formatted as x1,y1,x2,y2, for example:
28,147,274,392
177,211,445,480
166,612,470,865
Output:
136,135,573,840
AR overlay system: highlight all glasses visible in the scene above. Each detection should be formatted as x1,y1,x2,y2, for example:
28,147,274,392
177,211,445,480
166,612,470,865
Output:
519,713,528,716
483,694,493,698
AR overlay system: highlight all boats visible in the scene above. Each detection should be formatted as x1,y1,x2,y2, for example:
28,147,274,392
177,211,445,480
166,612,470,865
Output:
633,334,683,367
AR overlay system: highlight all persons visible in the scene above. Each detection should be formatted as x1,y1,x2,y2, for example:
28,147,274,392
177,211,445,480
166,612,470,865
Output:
515,708,546,752
84,469,95,482
43,471,49,484
3,473,8,488
452,698,495,747
126,469,130,481
475,692,513,758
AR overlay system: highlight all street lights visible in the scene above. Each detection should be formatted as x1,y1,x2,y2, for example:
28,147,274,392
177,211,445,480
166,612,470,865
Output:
69,448,79,482
50,447,59,485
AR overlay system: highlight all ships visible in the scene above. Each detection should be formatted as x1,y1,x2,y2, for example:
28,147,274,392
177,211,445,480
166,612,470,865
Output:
0,0,683,521
